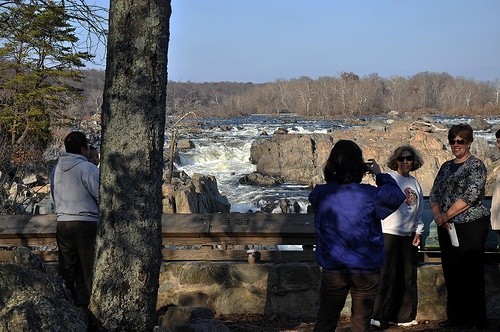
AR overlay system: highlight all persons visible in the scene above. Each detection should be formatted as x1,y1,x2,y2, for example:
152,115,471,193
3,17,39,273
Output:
308,139,407,332
369,146,425,327
428,123,489,332
88,146,99,166
50,131,99,305
490,130,500,231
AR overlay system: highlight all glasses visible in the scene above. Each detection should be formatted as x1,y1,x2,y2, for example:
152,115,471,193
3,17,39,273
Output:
397,156,414,162
449,139,465,145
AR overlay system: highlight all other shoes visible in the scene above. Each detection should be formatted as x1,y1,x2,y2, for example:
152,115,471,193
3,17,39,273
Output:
370,319,381,327
398,320,418,326
439,320,449,328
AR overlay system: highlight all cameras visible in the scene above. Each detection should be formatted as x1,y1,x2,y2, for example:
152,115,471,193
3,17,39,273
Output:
364,163,371,171
